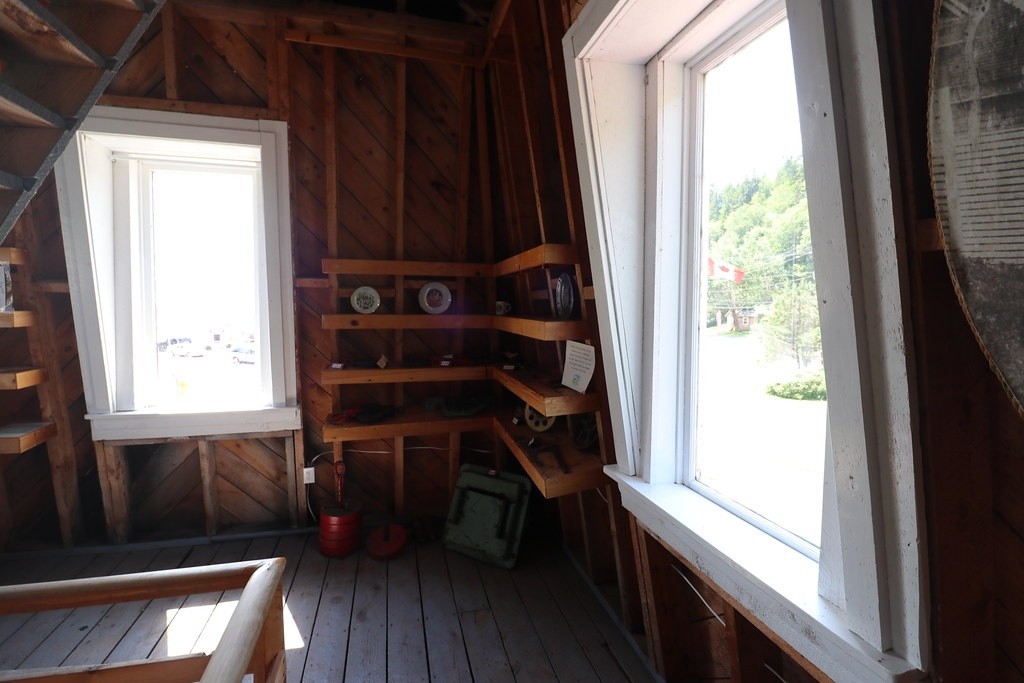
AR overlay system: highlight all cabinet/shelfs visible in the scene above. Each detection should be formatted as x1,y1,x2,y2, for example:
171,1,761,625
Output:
0,247,57,455
322,243,606,501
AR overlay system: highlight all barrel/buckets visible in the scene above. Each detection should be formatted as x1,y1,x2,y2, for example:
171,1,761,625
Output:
318,506,361,558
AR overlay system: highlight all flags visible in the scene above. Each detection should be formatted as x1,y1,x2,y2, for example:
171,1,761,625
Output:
708,258,744,283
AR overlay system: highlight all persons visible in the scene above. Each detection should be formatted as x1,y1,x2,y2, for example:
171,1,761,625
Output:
730,309,741,332
